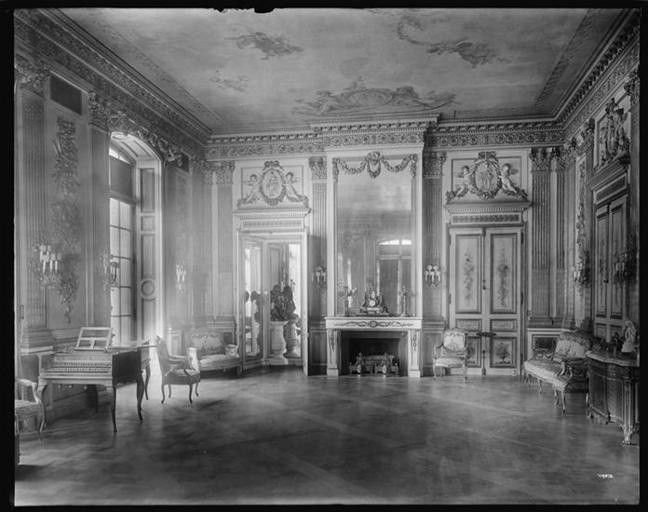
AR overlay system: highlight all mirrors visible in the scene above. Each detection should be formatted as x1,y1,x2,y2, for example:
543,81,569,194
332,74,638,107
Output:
267,241,303,358
244,237,269,361
335,158,414,316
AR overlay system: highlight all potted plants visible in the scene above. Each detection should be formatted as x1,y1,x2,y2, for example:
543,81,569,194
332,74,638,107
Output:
271,282,291,367
286,298,299,358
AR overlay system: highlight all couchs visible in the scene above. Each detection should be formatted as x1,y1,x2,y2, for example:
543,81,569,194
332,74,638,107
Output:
184,325,239,377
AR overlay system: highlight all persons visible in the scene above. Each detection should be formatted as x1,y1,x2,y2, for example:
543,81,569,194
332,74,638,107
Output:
498,163,518,195
455,166,475,199
243,174,262,203
283,173,300,203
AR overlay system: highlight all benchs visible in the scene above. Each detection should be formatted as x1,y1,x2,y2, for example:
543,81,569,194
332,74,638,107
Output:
523,328,591,414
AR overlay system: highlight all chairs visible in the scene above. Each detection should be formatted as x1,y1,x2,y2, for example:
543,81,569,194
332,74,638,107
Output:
432,327,471,382
153,334,199,403
15,374,50,468
